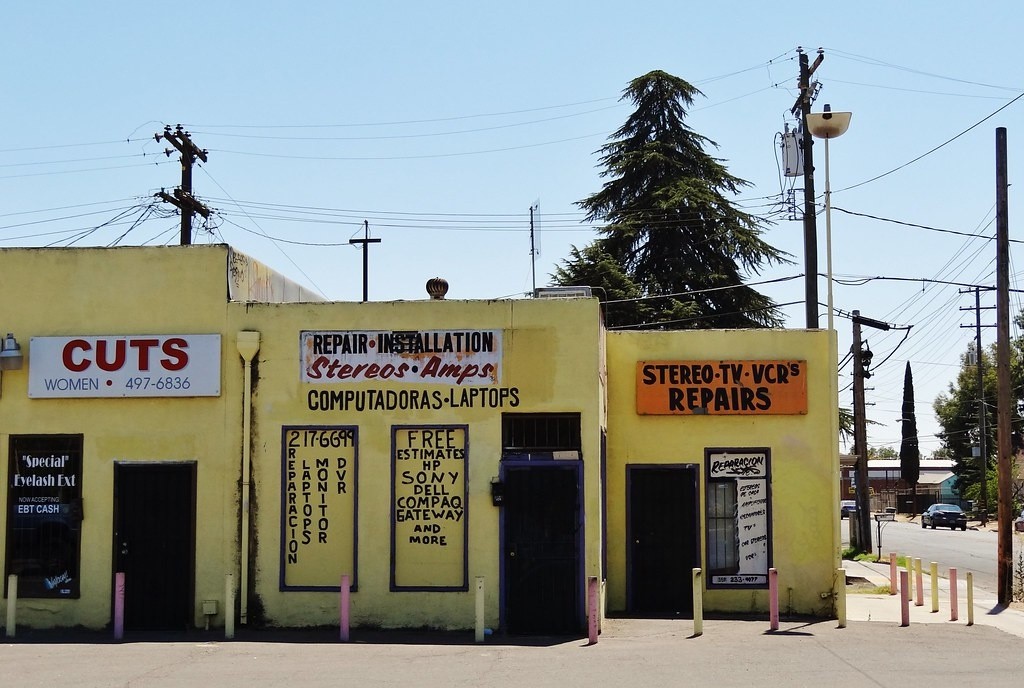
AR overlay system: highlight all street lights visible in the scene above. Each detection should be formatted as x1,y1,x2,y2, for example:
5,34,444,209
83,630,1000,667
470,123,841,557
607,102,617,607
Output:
807,105,853,333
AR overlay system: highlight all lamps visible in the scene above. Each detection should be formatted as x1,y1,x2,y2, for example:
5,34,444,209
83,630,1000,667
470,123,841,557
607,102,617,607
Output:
0,333,24,371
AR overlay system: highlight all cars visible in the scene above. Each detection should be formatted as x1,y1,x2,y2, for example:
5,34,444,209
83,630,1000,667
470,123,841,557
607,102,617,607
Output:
841,500,856,520
1014,510,1024,532
921,504,968,531
